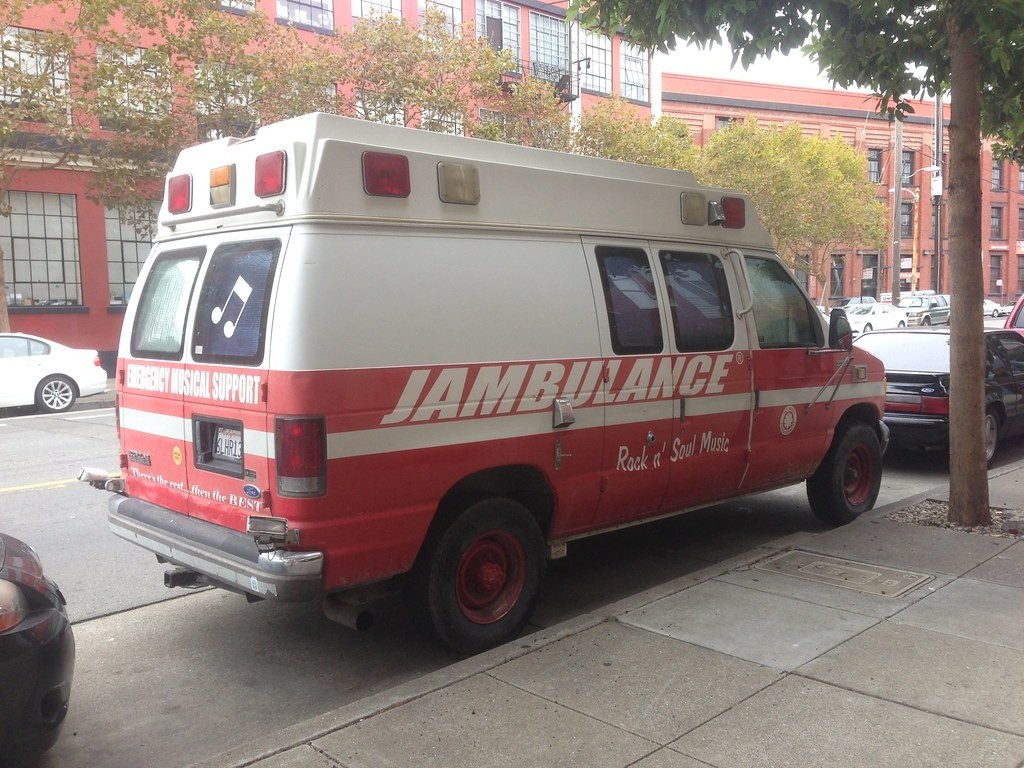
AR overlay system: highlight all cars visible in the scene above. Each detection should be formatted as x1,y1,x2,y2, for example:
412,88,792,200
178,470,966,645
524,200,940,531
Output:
0,332,109,416
1000,301,1017,316
851,326,1024,469
1,534,75,767
825,302,906,337
983,299,1002,318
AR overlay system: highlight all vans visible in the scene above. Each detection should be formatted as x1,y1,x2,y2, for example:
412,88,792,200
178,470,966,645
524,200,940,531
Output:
879,290,935,303
1003,293,1024,340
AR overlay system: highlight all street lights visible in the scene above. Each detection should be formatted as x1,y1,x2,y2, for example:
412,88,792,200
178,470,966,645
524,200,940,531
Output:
892,166,943,306
888,186,918,292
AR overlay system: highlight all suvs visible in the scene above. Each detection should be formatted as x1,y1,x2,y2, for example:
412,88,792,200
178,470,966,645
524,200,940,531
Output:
898,294,951,326
830,294,876,310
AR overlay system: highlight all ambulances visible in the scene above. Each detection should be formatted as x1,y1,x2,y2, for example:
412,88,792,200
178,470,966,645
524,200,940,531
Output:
75,106,891,652
